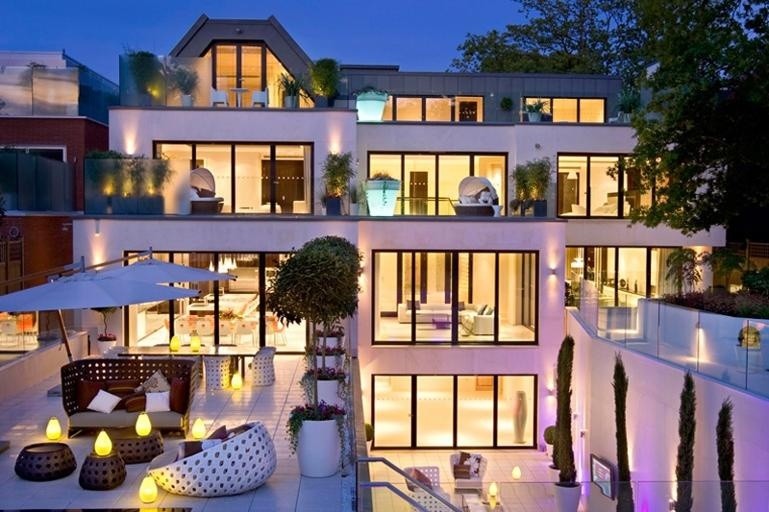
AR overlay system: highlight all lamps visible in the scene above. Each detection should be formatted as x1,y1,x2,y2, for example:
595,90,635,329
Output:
132,412,153,437
138,473,161,502
489,482,497,510
167,334,181,350
44,416,62,439
189,332,205,351
91,427,115,454
565,167,581,181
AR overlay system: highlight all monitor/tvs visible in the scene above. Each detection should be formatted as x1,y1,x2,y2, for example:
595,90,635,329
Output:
590,452,619,501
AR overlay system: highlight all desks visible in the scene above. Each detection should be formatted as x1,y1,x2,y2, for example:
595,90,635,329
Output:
229,85,250,108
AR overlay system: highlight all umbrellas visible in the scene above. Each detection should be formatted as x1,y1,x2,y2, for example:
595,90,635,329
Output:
0,255,201,362
62,247,238,286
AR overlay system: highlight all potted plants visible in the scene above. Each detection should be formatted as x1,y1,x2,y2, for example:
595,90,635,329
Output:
522,99,547,123
90,306,118,353
83,151,173,215
274,58,389,121
125,50,163,110
541,335,584,512
365,424,376,456
316,151,404,215
264,234,364,479
735,324,763,374
614,87,644,122
510,156,555,219
168,60,207,108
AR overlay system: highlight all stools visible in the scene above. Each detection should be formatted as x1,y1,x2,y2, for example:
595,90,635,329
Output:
14,424,165,492
432,317,452,330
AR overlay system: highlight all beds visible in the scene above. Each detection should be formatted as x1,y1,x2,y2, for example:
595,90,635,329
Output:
557,190,638,216
176,167,225,214
453,176,503,218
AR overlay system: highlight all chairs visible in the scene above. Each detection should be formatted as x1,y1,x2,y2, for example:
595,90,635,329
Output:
1,312,37,348
450,453,488,492
59,344,277,439
165,311,294,345
251,85,269,108
145,419,278,498
208,85,227,108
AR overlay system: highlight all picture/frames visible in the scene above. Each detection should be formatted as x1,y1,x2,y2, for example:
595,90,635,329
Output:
587,453,616,502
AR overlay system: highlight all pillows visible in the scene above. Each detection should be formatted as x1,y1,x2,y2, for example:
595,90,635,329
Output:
459,190,493,204
192,185,216,197
175,423,251,459
453,462,470,479
482,306,494,315
458,302,465,312
459,451,483,478
477,304,488,315
406,468,433,492
407,299,420,310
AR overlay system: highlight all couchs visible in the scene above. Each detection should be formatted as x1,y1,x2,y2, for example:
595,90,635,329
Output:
398,301,464,323
461,303,496,335
402,466,451,512
